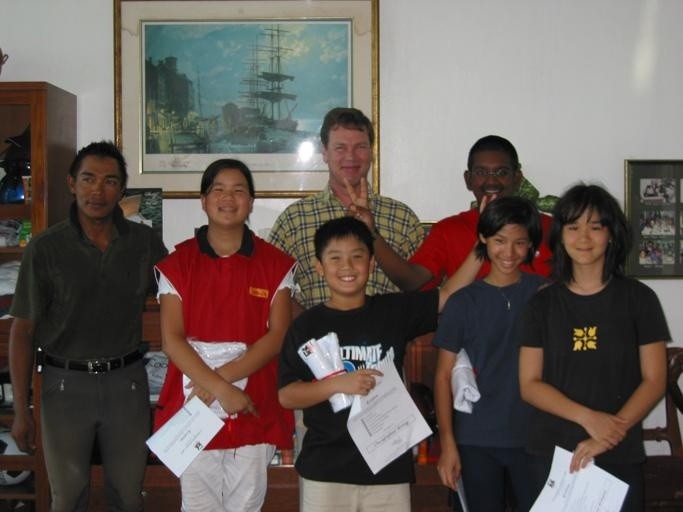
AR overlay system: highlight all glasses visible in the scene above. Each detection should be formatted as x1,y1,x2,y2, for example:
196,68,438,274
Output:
469,168,510,178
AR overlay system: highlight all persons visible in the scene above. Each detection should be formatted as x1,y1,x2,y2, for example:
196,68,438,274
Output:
8,143,170,512
432,197,554,512
266,108,426,319
330,135,554,293
154,158,299,512
277,194,497,512
519,184,672,511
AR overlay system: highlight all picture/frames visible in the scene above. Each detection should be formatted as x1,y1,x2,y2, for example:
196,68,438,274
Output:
621,158,683,281
113,0,381,200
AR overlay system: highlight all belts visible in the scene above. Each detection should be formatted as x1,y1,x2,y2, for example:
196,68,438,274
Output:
38,350,141,374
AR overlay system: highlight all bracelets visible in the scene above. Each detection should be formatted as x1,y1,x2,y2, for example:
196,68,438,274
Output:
371,227,379,241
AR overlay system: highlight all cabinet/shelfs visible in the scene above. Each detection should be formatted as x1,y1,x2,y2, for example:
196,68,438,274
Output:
0,80,78,512
